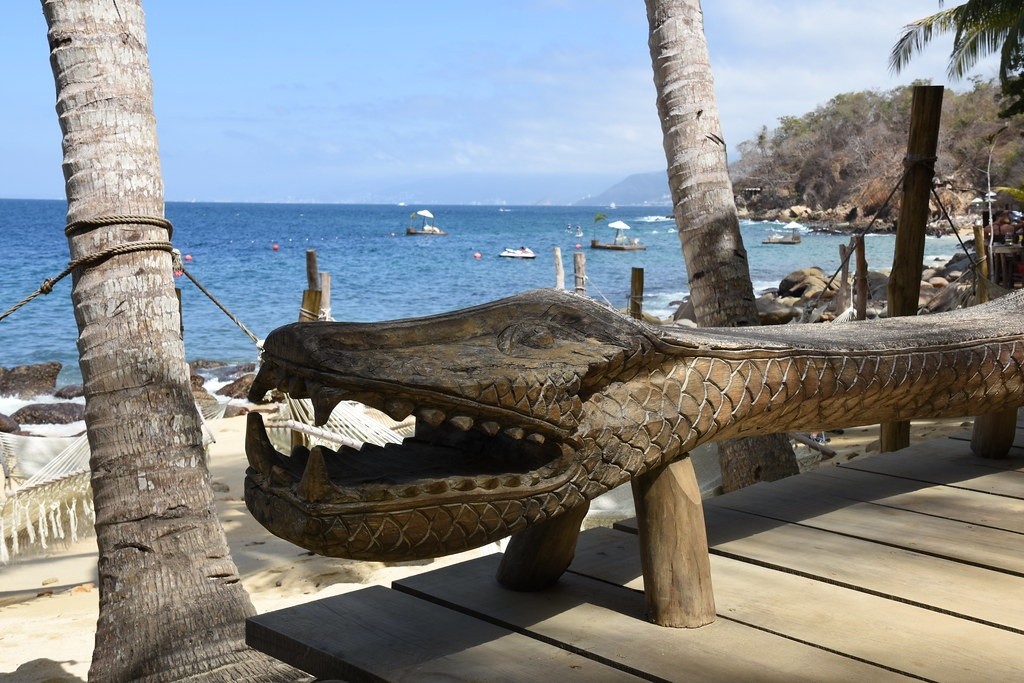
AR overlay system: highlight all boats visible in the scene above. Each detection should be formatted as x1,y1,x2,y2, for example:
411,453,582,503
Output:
406,209,447,235
590,213,647,250
398,202,408,207
499,246,536,259
762,220,803,244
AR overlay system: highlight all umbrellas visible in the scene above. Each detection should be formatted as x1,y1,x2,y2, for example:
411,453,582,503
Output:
608,220,630,245
417,210,433,231
971,191,997,206
784,221,803,234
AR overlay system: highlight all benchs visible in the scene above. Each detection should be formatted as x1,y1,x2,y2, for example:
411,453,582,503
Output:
244,287,1023,629
